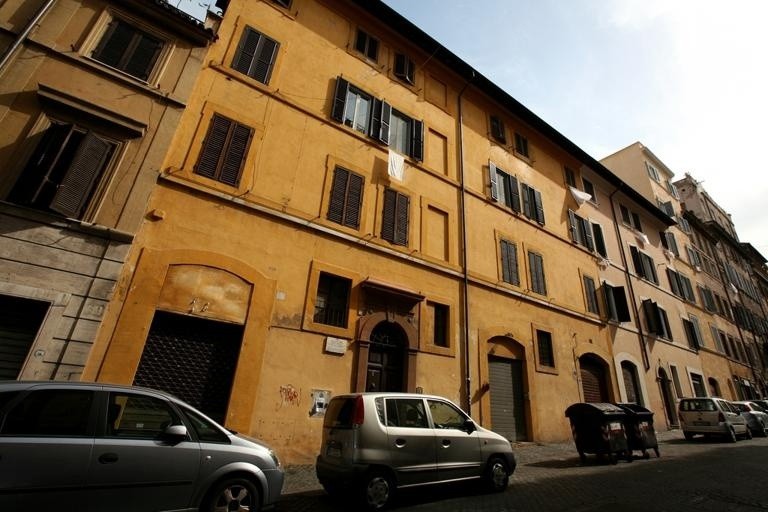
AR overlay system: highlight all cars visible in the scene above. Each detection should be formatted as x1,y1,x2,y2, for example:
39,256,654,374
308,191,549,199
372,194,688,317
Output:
315,391,516,512
678,397,768,443
0,380,285,511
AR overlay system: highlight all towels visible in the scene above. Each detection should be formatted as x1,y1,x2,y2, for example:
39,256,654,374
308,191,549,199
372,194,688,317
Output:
387,148,405,181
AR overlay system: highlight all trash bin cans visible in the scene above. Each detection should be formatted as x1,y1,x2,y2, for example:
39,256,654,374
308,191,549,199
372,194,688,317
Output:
565,402,660,464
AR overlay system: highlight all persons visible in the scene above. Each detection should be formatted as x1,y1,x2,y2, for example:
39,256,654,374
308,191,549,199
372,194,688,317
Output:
405,409,421,428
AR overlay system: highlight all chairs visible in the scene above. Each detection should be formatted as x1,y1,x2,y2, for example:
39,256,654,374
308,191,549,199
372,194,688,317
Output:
408,408,419,422
109,403,121,432
